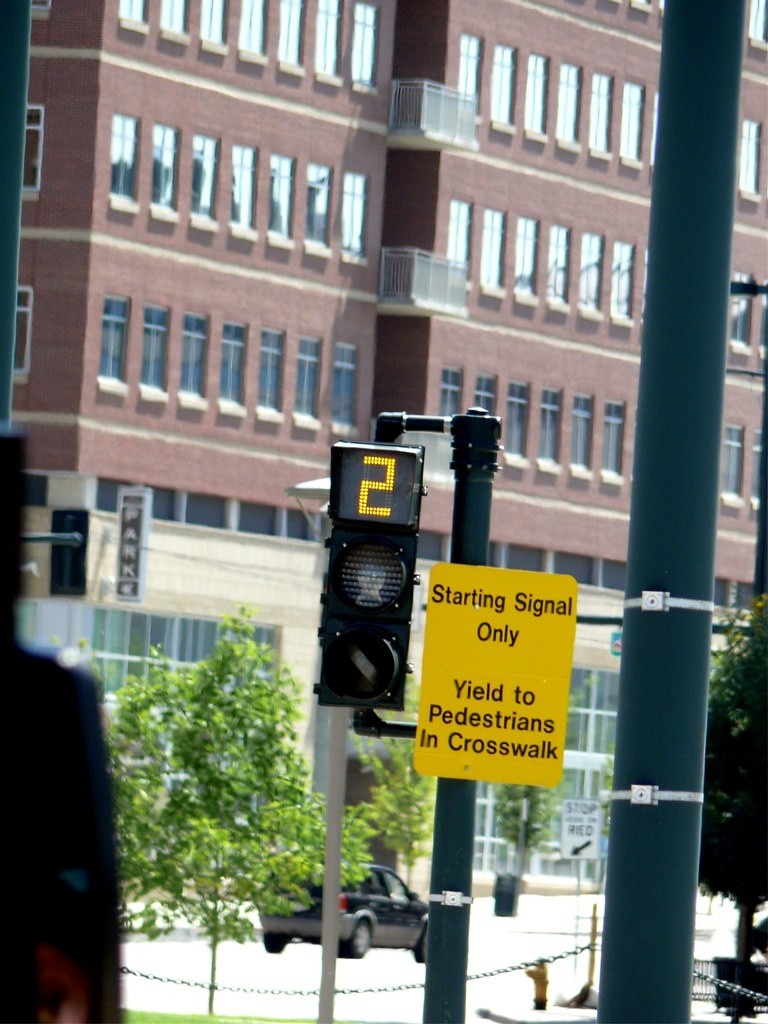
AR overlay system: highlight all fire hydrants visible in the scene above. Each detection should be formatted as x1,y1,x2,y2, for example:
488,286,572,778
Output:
525,958,548,1010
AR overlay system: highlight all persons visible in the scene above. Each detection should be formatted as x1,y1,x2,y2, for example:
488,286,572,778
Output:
0,430,126,1024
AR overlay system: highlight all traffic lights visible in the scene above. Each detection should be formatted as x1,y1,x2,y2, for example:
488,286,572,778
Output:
324,437,431,534
313,528,417,714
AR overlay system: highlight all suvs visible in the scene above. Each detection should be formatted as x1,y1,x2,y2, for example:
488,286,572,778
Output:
258,864,430,962
